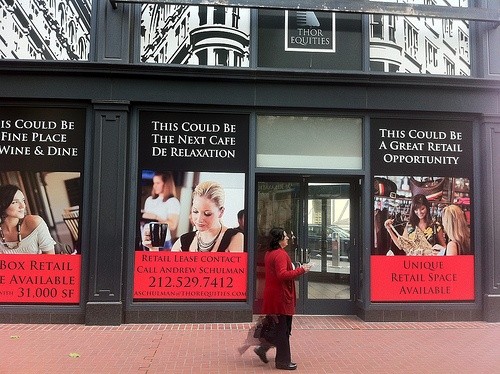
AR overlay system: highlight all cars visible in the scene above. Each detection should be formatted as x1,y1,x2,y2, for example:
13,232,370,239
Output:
307,223,350,262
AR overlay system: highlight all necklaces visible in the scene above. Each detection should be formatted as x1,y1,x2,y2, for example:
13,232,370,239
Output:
196,222,222,252
0,219,23,250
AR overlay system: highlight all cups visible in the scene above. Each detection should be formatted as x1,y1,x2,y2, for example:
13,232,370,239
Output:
149,222,168,247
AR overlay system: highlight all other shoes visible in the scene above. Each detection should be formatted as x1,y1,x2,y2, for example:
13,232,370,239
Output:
276,361,297,370
253,347,269,363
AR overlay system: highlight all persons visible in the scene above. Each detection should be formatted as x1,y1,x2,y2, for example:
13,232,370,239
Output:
254,227,311,370
140,171,181,251
441,205,470,256
233,209,244,235
384,194,446,256
142,180,244,253
0,184,56,254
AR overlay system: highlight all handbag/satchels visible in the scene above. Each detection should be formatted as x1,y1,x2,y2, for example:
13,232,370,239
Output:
245,314,275,348
